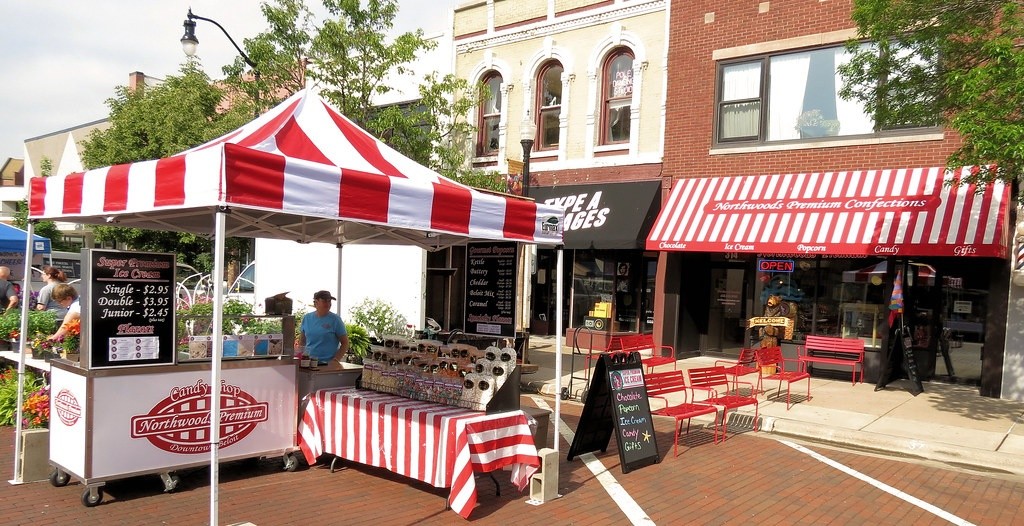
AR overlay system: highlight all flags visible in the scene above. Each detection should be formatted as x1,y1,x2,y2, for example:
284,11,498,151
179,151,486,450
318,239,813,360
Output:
889,271,903,328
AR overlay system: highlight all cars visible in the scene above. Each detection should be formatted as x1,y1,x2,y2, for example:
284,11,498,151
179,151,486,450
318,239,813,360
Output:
176,262,215,304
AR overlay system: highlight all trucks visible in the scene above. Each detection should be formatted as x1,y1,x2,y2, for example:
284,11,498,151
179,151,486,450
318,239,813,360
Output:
220,232,466,341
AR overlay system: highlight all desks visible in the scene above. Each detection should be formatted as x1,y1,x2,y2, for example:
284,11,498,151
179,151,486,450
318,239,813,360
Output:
296,386,540,519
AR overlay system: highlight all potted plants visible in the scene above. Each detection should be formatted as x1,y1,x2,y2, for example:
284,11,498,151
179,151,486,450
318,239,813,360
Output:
0,307,58,430
793,108,826,139
821,119,841,136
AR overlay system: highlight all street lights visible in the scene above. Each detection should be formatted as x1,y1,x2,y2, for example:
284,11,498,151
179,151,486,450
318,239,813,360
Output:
180,6,259,119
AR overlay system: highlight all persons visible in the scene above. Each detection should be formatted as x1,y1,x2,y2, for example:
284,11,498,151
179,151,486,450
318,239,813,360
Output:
0,266,18,315
48,285,81,340
37,266,67,330
300,291,348,366
619,263,628,275
617,279,628,292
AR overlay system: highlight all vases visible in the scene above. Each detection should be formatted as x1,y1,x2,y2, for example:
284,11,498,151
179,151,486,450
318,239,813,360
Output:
31,347,44,359
44,348,59,363
66,352,80,362
10,340,33,353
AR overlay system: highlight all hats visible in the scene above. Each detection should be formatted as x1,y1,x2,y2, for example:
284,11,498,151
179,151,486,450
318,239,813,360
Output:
314,291,336,301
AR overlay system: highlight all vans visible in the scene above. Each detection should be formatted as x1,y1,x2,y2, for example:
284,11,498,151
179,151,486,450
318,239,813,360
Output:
12,251,82,298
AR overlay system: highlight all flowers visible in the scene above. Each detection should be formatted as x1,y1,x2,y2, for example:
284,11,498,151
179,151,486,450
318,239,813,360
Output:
31,331,49,349
9,326,33,341
40,336,64,351
10,383,50,433
60,318,82,354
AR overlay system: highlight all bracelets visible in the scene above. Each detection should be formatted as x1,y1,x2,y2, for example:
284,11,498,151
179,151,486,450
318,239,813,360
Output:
333,358,340,364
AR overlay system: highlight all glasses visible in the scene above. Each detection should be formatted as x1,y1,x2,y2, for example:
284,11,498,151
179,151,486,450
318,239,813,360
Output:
58,297,67,305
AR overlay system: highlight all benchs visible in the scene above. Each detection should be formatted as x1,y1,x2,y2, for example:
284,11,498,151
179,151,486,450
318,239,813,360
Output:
755,348,810,410
713,346,768,398
687,365,758,441
584,332,644,380
620,334,677,378
797,335,864,386
643,370,718,459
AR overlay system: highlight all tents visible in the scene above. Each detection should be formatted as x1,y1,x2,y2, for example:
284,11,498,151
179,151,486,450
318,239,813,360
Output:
836,261,936,336
0,222,52,273
8,87,565,526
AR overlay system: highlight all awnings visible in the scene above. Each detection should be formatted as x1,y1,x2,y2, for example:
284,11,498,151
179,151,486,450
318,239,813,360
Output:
646,163,1011,259
526,181,662,251
